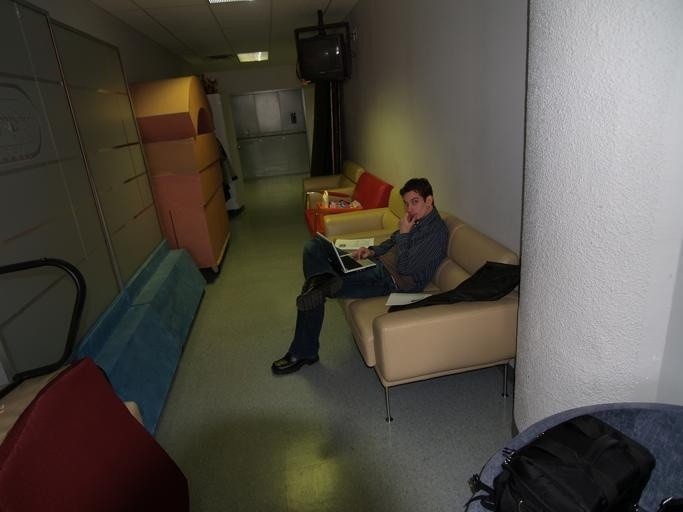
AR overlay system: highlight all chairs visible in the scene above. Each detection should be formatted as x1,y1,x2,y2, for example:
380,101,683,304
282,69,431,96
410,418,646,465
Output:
303,160,365,195
465,403,681,510
303,172,393,237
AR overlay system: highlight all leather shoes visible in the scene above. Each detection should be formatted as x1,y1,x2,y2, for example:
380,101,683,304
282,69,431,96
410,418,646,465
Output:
295,273,343,312
271,351,319,375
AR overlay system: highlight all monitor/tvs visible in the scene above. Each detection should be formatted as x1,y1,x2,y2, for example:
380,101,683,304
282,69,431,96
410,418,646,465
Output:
298,34,352,83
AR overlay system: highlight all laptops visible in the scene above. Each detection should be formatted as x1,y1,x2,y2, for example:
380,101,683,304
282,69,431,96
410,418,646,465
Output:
317,231,376,273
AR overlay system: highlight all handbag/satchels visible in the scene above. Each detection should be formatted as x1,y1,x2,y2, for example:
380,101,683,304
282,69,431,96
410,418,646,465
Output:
463,412,657,511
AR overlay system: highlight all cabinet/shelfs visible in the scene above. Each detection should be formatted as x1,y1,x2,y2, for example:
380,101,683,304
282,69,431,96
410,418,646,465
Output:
132,76,232,279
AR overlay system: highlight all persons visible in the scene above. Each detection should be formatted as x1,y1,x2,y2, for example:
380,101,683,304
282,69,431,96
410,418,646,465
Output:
269,177,448,373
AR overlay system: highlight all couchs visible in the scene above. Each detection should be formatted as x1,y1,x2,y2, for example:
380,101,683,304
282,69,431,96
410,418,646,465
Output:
324,188,518,423
